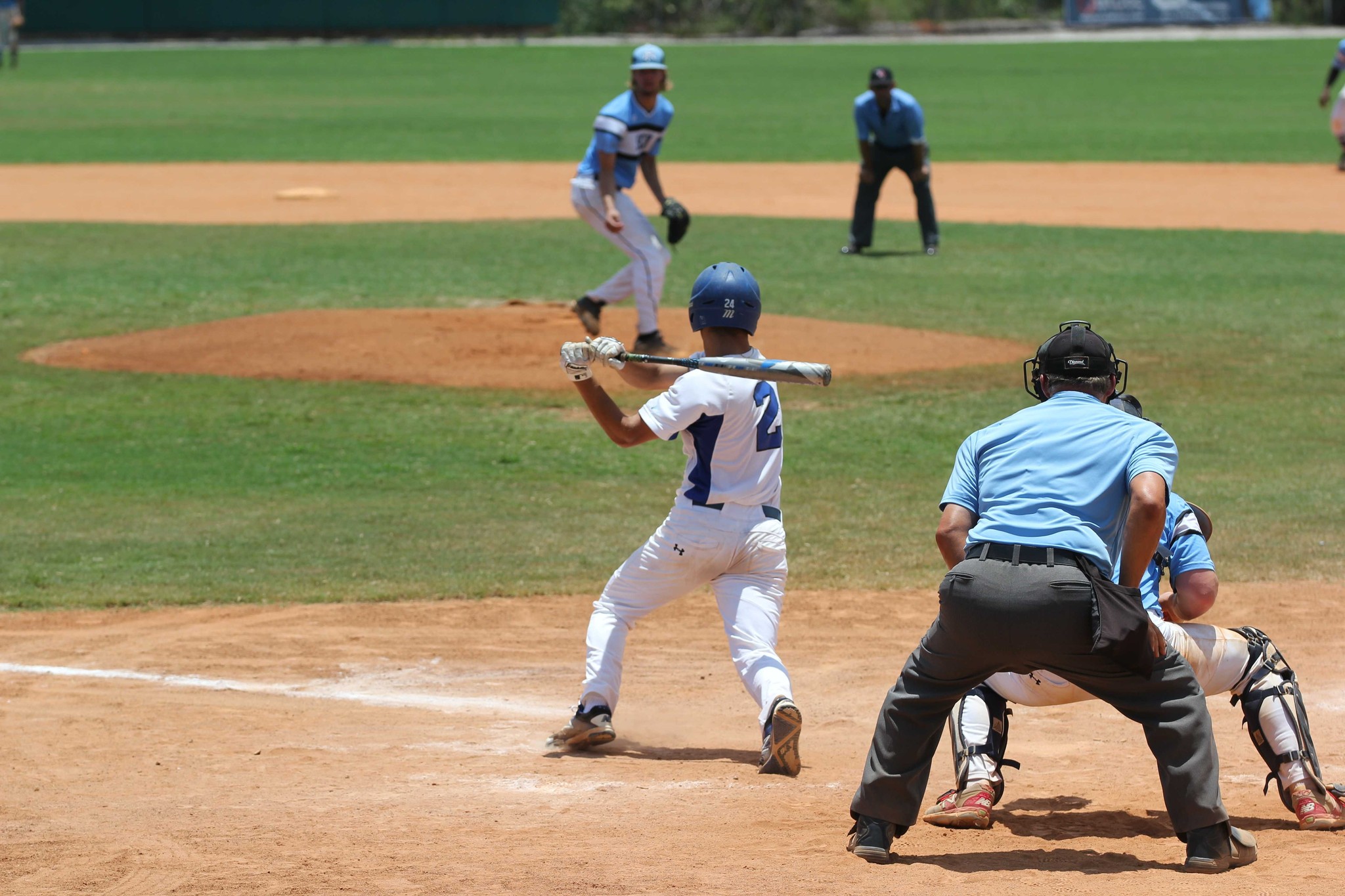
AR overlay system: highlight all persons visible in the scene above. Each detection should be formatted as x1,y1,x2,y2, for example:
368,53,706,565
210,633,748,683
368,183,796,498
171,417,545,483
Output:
1319,38,1345,171
544,263,801,777
841,65,941,256
845,316,1256,876
568,43,692,358
921,392,1345,832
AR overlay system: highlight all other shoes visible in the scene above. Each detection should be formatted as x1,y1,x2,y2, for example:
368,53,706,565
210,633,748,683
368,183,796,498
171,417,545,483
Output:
842,242,861,254
634,332,675,355
569,294,606,335
925,244,938,255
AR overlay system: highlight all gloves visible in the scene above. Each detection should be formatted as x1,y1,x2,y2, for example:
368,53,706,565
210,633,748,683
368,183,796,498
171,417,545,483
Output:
589,336,627,370
559,336,593,382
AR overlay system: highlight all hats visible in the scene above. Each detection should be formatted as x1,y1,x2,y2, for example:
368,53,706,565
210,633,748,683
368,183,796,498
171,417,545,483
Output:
1037,326,1117,378
868,67,893,88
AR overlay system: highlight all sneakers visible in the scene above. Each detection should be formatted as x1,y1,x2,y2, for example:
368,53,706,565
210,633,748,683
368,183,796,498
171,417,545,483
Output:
1291,788,1345,829
546,701,616,752
1183,822,1258,874
758,697,802,776
845,814,896,864
923,782,995,828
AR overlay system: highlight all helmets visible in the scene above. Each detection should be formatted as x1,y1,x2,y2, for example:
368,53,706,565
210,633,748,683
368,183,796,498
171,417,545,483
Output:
688,262,761,336
630,43,668,71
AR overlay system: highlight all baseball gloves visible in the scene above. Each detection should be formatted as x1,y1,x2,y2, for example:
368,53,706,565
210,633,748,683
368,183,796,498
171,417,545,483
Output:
658,199,692,244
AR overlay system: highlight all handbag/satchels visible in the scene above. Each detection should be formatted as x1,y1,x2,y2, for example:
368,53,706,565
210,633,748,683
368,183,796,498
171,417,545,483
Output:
1072,557,1148,651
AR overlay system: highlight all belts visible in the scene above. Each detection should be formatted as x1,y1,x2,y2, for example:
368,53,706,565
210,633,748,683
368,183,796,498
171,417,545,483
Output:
701,502,781,521
968,543,1081,567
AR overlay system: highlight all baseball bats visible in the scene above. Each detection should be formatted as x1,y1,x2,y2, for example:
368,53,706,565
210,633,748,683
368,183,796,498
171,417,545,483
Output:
614,351,834,388
